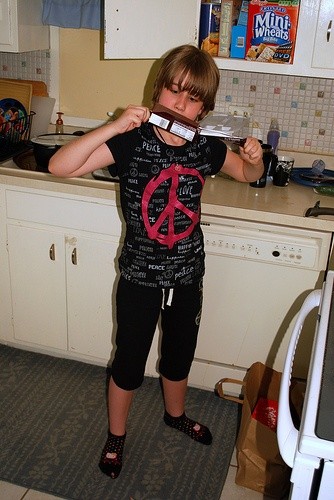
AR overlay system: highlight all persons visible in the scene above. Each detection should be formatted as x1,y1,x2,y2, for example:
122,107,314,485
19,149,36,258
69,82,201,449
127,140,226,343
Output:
48,45,265,479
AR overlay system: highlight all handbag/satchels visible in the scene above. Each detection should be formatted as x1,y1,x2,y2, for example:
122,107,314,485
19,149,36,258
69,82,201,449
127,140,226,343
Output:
215,362,305,500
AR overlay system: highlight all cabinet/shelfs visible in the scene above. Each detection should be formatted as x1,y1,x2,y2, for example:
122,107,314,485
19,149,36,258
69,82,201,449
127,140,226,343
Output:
0,150,334,401
103,0,334,80
0,0,50,54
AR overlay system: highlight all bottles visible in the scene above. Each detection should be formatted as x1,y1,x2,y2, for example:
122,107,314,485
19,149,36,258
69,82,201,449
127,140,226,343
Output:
267,119,281,155
56,111,64,133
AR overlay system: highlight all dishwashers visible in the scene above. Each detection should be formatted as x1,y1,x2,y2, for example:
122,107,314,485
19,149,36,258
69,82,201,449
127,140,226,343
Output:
199,214,332,398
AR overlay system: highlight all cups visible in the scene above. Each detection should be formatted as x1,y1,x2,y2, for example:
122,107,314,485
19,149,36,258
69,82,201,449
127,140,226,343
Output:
249,144,274,188
273,156,295,187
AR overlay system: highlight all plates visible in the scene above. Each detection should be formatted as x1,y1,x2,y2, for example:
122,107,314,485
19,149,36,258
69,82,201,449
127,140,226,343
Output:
31,135,80,146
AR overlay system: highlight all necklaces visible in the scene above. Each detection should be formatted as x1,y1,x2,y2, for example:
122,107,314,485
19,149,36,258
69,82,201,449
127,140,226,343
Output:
155,125,188,171
